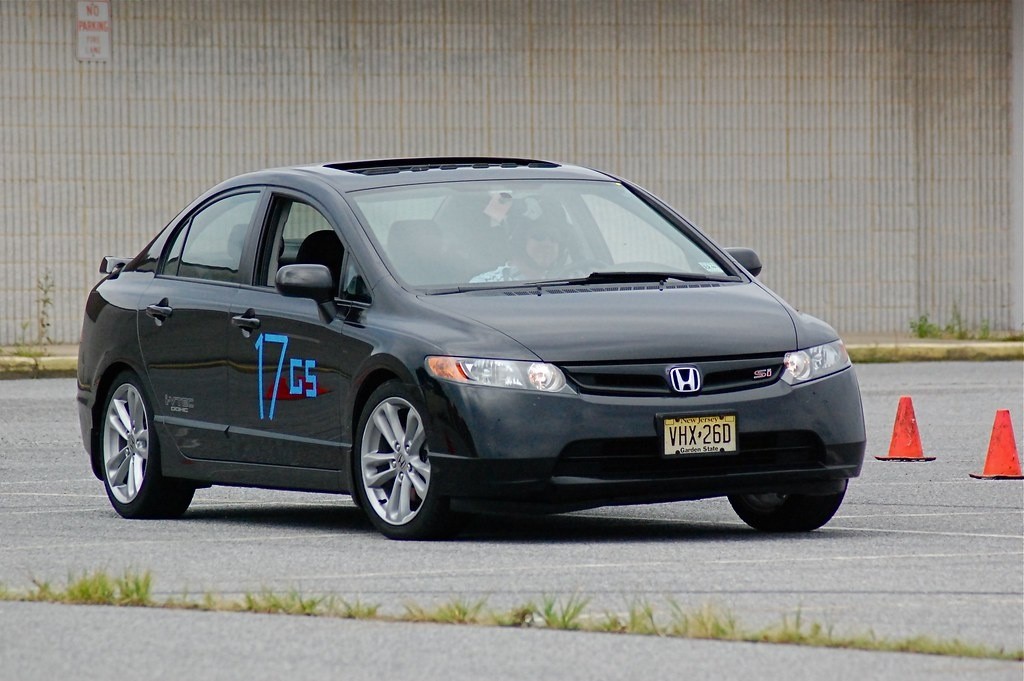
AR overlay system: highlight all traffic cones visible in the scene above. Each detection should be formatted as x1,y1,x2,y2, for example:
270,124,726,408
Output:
874,395,938,461
968,410,1024,481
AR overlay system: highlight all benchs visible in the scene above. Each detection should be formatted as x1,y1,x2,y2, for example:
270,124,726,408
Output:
189,220,503,281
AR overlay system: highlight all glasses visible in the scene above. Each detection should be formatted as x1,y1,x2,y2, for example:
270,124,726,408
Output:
529,231,564,243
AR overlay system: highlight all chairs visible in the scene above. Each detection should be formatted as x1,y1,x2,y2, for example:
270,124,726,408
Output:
297,231,343,280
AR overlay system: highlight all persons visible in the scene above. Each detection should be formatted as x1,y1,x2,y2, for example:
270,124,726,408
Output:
466,195,595,283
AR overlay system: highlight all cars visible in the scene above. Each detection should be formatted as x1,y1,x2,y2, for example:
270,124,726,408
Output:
74,156,866,544
